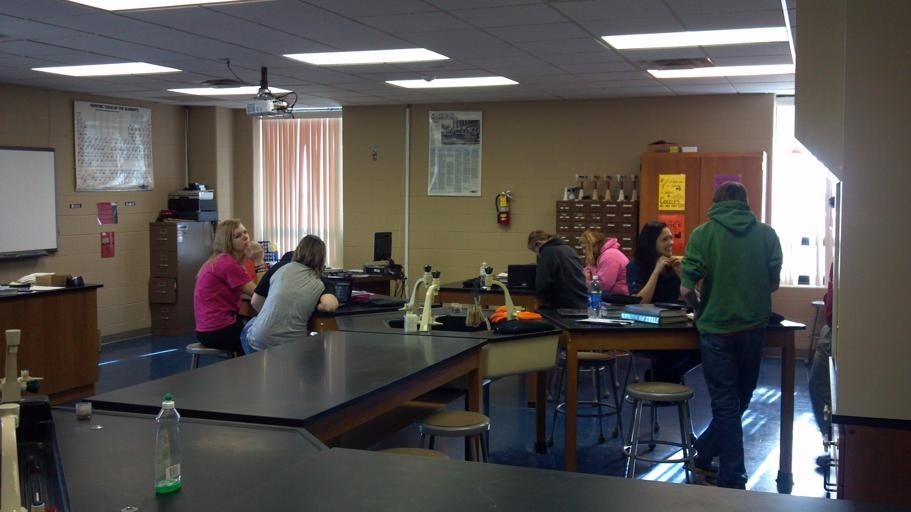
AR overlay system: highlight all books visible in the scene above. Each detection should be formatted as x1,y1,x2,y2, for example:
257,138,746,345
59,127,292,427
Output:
599,302,691,325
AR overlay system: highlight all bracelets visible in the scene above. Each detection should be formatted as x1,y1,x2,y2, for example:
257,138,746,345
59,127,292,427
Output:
254,263,269,272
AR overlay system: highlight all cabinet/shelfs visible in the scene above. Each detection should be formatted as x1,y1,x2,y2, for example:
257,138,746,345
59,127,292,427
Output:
559,196,635,269
148,221,217,335
639,148,769,269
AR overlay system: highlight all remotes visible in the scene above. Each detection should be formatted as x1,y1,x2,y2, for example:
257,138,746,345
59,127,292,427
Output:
654,302,685,310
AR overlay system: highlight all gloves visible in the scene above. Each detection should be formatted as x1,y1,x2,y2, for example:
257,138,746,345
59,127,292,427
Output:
489,305,541,323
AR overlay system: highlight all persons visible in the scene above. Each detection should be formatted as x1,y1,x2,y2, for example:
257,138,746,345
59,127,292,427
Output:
579,226,636,307
238,233,342,356
191,217,271,356
627,217,704,383
811,259,832,467
677,179,783,493
526,230,592,315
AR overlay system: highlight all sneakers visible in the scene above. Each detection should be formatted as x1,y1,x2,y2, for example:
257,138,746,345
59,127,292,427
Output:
816,455,833,467
703,475,746,490
683,455,722,475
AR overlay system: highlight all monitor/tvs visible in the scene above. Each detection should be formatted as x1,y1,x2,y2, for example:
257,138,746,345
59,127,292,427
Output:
508,264,536,291
373,232,397,274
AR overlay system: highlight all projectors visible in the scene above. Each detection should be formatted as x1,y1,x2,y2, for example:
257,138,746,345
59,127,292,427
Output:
246,88,295,120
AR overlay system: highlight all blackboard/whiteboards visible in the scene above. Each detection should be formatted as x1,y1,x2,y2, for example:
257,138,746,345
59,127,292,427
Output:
0,145,58,257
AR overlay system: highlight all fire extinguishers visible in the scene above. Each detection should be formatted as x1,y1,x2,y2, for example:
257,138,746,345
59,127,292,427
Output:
496,190,513,225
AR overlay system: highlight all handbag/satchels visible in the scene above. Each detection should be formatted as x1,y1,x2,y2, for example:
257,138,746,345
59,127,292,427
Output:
602,292,642,304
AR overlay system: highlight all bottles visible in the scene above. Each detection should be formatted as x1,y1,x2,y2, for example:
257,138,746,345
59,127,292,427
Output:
153,392,183,494
589,274,602,319
